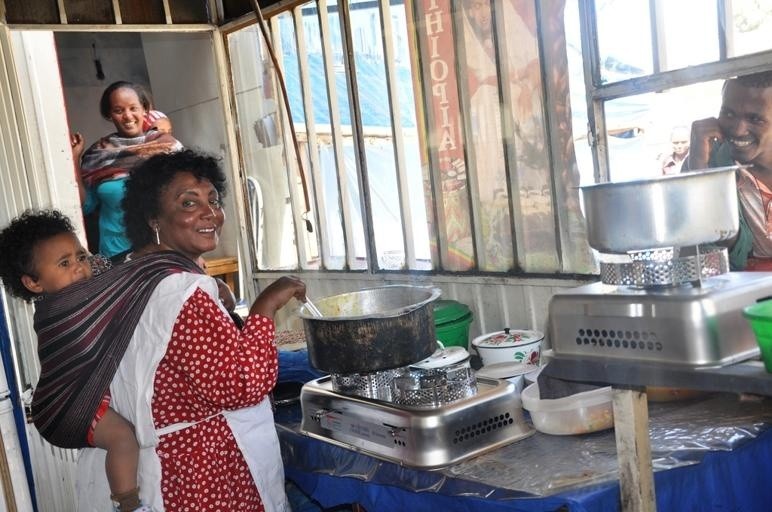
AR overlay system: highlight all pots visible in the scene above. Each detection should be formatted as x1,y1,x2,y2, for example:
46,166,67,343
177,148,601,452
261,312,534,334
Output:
562,160,745,253
297,284,437,374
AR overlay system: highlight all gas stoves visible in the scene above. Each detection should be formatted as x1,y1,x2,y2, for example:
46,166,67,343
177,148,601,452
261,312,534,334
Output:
298,368,533,472
548,248,771,361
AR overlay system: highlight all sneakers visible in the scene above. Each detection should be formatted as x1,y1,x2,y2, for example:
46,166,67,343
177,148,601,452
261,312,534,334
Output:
116,505,153,512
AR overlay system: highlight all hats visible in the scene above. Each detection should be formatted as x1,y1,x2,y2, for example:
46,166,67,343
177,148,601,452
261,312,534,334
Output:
143,110,168,132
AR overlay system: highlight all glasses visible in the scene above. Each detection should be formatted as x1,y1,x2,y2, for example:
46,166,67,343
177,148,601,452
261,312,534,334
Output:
673,140,689,144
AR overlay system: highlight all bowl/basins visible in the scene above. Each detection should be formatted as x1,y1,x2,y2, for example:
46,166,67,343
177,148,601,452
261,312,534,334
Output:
470,330,546,366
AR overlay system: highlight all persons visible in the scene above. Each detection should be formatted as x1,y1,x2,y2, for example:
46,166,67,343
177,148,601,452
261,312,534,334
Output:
70,79,188,265
107,145,307,512
680,70,772,271
1,209,159,512
142,109,172,134
661,126,690,175
